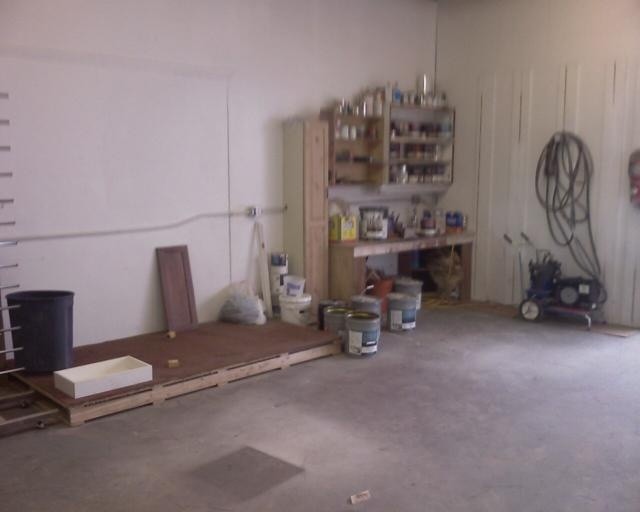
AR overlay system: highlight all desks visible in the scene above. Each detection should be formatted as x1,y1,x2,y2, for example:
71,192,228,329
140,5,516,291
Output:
328,225,475,305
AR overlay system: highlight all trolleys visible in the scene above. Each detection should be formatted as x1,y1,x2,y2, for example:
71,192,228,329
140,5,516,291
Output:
504,231,605,331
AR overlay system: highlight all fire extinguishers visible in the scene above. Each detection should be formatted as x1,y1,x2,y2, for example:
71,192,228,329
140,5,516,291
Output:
629,148,640,204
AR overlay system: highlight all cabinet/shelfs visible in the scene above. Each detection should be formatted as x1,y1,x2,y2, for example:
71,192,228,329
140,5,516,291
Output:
280,114,331,324
319,97,458,193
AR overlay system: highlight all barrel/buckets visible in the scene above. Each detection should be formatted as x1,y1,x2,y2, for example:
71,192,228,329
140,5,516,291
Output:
350,285,382,314
323,305,353,333
386,292,417,332
342,310,381,358
367,277,394,315
278,292,313,324
283,275,306,297
319,298,347,328
5,290,74,375
393,279,423,311
269,253,290,294
